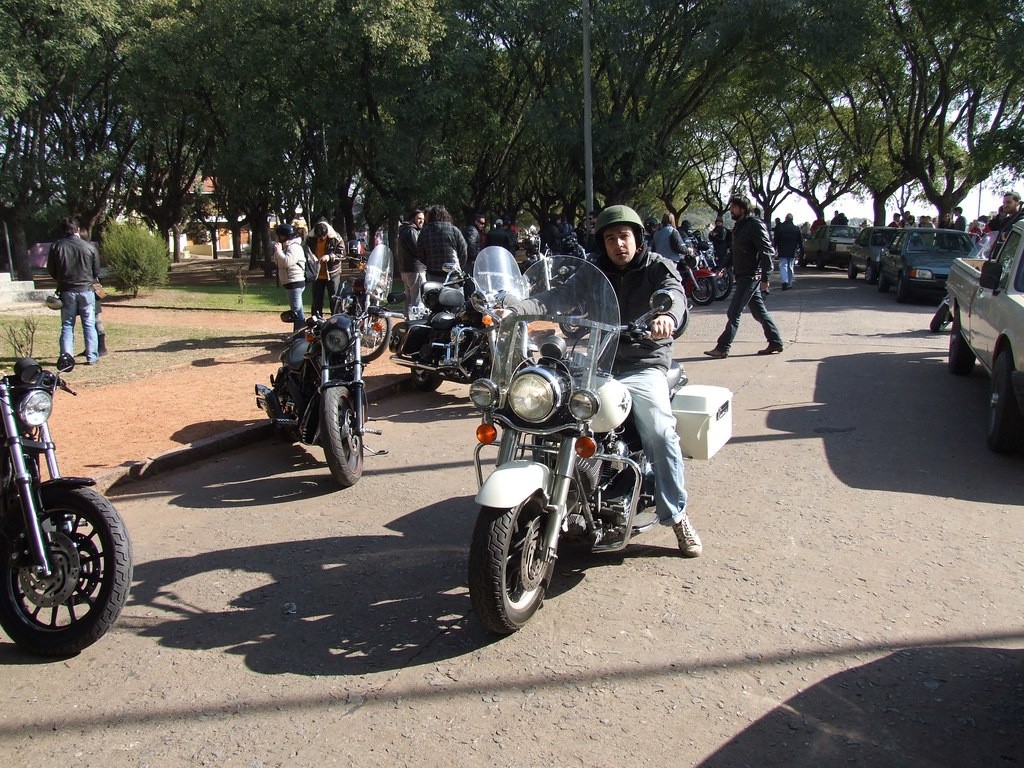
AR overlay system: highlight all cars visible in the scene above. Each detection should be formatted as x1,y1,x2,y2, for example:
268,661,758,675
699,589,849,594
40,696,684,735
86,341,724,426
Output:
848,226,978,303
803,225,864,271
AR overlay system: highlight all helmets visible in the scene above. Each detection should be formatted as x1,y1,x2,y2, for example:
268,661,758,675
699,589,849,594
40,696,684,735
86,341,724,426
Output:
594,204,645,241
275,224,295,235
46,295,62,309
644,217,659,236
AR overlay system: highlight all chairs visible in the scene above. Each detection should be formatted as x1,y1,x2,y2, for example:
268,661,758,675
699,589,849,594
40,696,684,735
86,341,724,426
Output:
911,236,925,246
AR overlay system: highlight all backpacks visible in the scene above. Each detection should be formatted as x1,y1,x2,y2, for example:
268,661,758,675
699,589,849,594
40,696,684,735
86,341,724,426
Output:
289,242,321,282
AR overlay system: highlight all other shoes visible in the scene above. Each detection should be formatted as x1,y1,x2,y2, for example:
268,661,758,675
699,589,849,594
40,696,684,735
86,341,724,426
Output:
781,282,787,291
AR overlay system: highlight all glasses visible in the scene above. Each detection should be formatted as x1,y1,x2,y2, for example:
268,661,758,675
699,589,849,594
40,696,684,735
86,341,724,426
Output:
476,219,485,226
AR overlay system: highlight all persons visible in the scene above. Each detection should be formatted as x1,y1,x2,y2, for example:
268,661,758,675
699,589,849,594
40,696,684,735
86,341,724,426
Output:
918,215,939,247
46,216,109,367
774,214,804,290
305,219,346,319
642,216,660,253
888,211,917,227
970,214,994,258
585,210,601,252
703,193,783,358
830,210,849,226
417,203,467,283
988,191,1024,259
463,214,518,302
938,206,966,249
398,209,426,322
265,220,309,333
801,214,826,239
708,217,732,259
676,220,693,239
653,212,693,311
491,205,703,558
544,212,585,253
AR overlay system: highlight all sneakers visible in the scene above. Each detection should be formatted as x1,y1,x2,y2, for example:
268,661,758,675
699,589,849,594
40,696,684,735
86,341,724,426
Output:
757,342,783,354
703,346,728,358
674,514,703,556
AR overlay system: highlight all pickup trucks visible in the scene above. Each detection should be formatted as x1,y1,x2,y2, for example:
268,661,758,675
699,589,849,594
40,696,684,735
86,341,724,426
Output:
930,219,1024,453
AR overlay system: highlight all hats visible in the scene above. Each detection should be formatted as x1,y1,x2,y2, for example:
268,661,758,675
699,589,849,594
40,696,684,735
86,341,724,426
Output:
715,217,723,224
495,219,503,225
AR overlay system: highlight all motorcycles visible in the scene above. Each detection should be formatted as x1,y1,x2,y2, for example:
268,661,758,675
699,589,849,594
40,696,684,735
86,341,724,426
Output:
678,223,734,306
255,244,407,487
0,352,134,662
389,247,659,634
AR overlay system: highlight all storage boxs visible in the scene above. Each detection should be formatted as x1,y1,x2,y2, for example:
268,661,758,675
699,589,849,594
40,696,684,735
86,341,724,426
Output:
671,384,735,461
390,319,431,354
